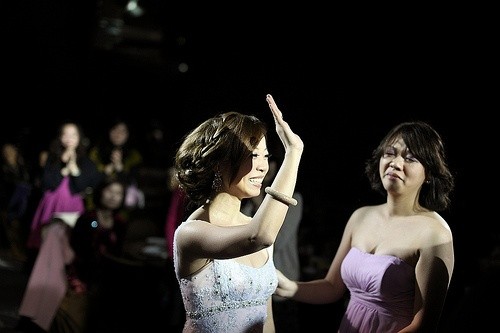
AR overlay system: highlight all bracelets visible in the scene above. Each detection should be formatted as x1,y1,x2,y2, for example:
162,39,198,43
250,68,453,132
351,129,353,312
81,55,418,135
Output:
264,186,297,207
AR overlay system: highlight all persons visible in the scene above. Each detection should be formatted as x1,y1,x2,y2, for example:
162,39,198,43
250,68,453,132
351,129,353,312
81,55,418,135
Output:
274,123,455,333
0,116,302,333
173,94,304,333
